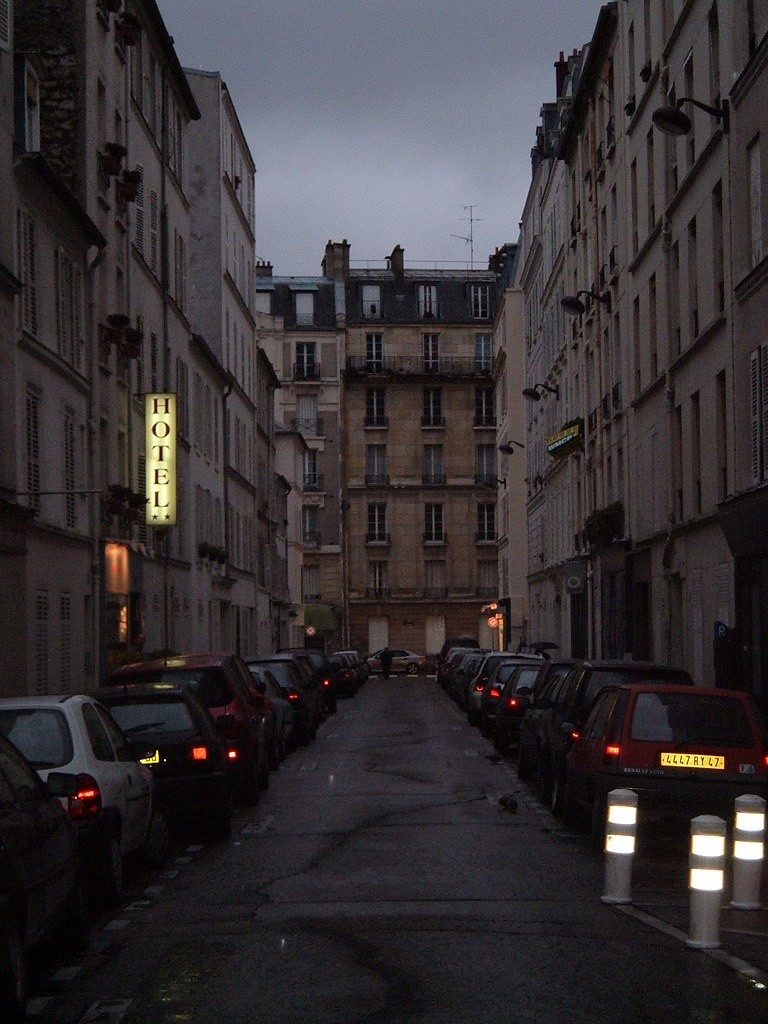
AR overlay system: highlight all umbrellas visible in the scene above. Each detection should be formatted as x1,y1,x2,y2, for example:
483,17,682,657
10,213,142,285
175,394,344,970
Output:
528,641,561,650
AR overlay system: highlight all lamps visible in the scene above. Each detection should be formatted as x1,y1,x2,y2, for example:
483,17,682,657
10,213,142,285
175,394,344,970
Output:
686,813,728,948
482,477,506,490
652,97,731,136
499,440,525,455
600,789,640,904
522,383,560,402
561,290,611,315
730,794,768,910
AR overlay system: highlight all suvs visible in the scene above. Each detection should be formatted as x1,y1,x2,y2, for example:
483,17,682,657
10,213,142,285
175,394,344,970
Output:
516,659,697,816
108,651,276,812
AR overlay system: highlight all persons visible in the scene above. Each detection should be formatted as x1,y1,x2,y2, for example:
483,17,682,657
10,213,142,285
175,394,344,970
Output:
534,649,551,660
380,647,394,679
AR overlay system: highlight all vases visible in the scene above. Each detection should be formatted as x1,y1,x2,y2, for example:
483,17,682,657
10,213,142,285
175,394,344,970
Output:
119,24,139,46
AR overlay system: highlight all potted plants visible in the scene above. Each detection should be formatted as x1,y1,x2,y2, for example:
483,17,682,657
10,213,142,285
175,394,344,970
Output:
105,313,131,344
119,169,144,203
124,328,144,359
103,483,136,515
100,142,128,177
118,11,143,36
198,541,209,558
126,493,150,521
217,549,229,565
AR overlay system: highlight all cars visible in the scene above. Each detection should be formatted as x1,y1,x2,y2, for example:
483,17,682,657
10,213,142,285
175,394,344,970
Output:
0,732,99,1024
1,692,170,904
560,680,768,838
436,636,587,758
366,648,431,676
85,681,233,843
240,648,368,772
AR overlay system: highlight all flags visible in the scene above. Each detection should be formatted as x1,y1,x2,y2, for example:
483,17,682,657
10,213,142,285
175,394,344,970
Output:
565,563,587,594
